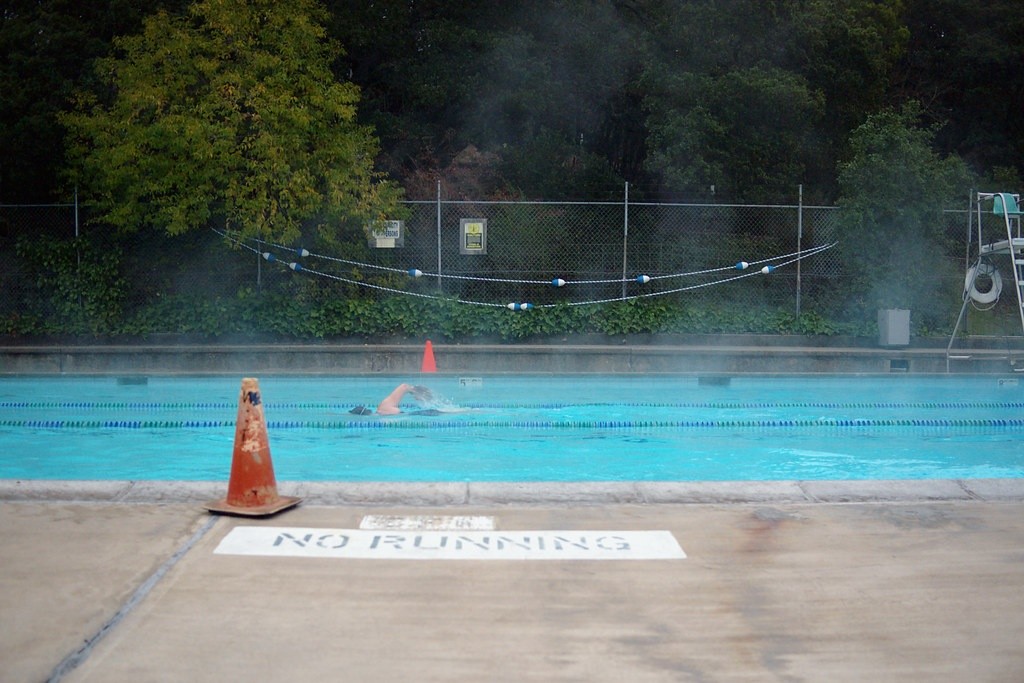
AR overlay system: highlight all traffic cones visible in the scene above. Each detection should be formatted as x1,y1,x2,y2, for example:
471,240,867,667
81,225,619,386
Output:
200,377,303,517
421,339,439,374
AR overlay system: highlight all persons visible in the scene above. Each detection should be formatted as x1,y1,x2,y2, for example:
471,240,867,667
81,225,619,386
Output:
351,383,416,416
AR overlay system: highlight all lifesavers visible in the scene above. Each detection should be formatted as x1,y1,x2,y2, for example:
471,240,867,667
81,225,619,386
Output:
964,263,1002,304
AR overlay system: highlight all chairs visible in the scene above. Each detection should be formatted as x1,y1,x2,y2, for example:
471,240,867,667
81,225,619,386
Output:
993,192,1024,238
